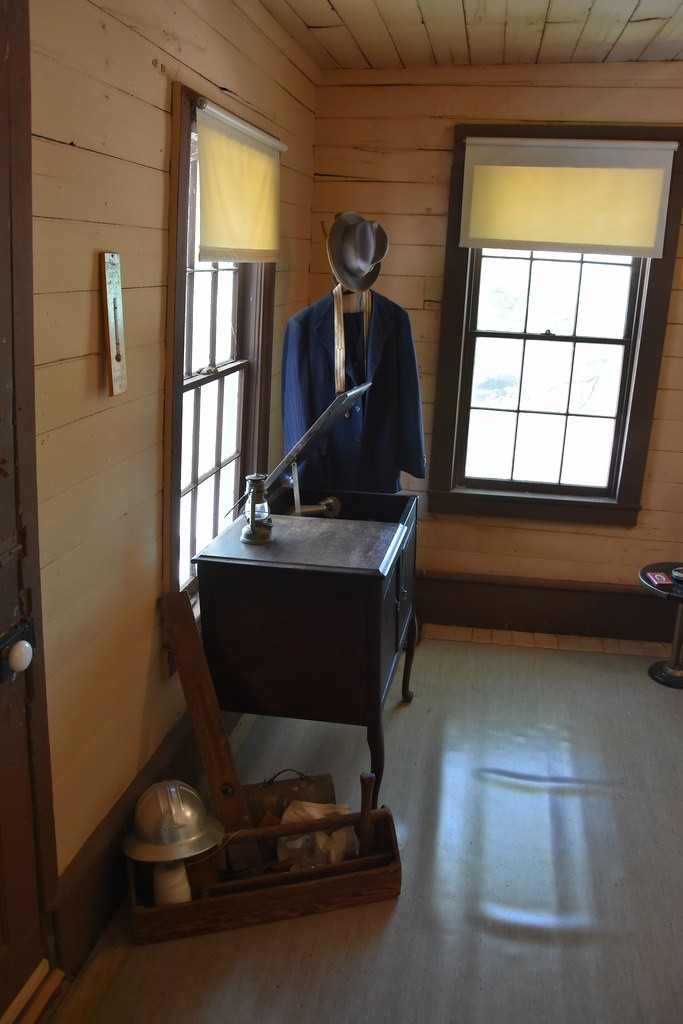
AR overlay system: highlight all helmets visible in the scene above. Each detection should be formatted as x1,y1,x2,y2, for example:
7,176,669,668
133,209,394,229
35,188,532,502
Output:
123,779,225,861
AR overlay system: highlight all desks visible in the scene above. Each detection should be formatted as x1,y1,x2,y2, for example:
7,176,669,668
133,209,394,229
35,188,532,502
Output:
638,562,683,689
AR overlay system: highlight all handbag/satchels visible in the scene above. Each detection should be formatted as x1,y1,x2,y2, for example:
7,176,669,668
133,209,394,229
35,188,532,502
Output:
242,769,336,827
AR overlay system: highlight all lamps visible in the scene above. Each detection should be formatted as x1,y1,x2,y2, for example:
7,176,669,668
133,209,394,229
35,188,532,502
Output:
224,473,275,545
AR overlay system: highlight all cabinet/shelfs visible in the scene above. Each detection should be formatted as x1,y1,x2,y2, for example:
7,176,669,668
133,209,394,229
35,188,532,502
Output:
189,384,419,812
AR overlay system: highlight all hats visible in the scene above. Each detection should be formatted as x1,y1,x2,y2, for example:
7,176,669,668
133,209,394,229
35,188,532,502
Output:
326,212,389,293
